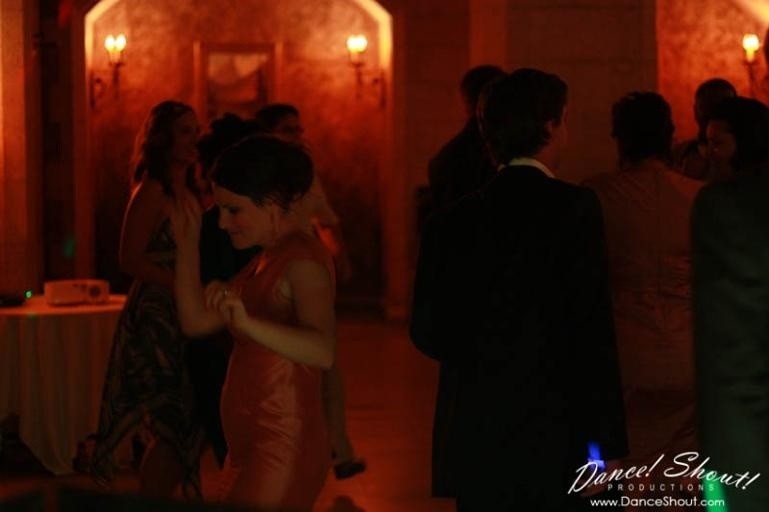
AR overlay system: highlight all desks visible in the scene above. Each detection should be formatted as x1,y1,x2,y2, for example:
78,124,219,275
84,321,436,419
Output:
0,292,128,478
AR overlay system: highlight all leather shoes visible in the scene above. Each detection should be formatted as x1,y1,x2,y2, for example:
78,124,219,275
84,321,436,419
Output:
335,461,365,479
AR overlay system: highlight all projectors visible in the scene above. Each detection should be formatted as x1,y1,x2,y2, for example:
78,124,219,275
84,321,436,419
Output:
45,279,109,306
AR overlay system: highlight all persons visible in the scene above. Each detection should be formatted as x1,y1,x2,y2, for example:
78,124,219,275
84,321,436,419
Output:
688,95,768,512
409,68,630,512
670,77,737,182
583,90,711,512
0,100,367,511
427,63,505,189
